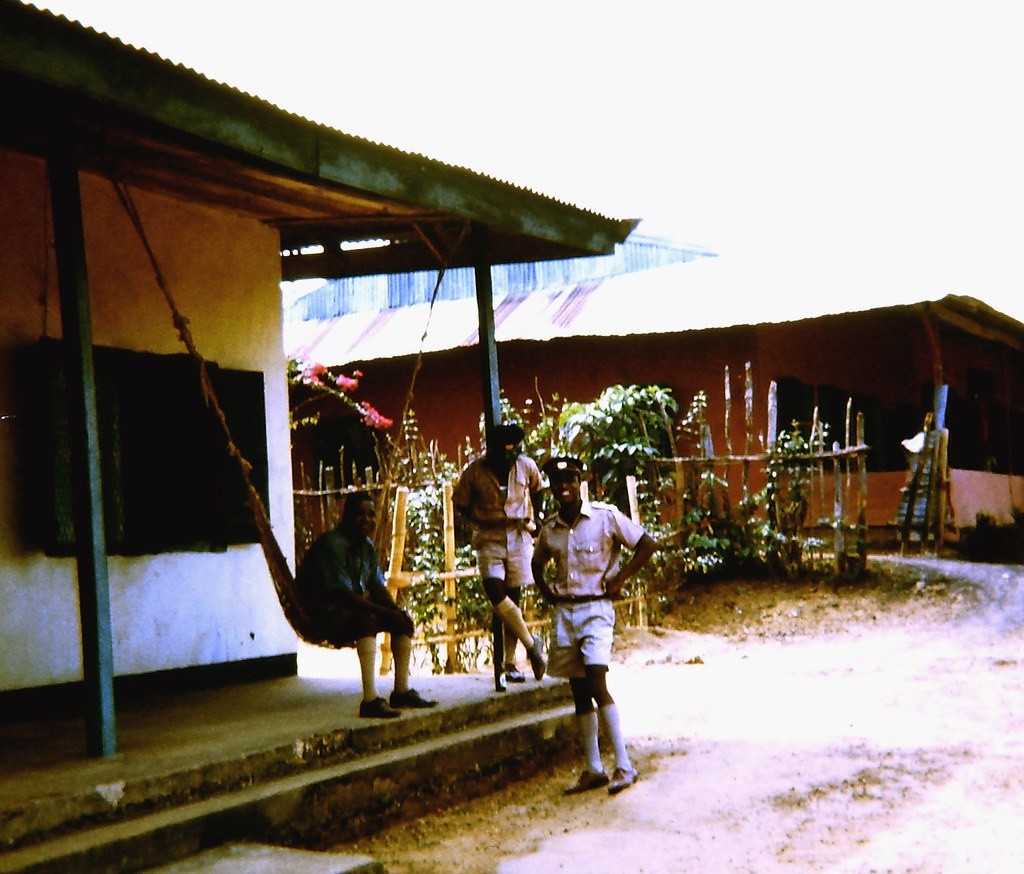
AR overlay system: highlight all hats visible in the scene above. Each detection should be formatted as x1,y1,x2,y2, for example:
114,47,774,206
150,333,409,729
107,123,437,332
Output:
542,457,583,476
487,425,524,452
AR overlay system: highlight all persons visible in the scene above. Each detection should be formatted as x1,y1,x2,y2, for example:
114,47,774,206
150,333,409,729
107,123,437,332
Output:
452,425,546,683
295,493,440,719
531,459,658,793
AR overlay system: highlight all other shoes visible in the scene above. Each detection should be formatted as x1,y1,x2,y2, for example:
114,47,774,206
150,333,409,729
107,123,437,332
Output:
564,771,609,794
360,698,399,718
609,765,638,794
527,635,546,680
390,689,437,708
505,664,525,682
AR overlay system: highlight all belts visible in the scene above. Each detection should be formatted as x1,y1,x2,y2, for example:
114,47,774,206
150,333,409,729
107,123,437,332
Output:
560,595,606,602
